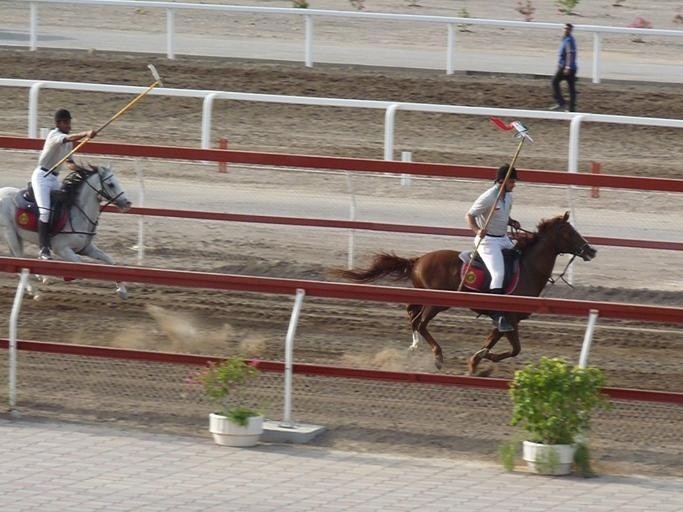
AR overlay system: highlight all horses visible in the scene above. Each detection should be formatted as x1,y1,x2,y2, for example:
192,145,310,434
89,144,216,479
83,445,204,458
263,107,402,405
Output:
0,159,133,302
320,211,598,377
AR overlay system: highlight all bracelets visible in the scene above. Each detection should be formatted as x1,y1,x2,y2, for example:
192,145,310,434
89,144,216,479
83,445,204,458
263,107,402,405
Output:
565,66,571,70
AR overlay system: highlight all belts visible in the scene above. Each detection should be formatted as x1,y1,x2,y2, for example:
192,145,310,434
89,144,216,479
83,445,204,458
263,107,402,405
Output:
484,234,507,239
41,167,59,176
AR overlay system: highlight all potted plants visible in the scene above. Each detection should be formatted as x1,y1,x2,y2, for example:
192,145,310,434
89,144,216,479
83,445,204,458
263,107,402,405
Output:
509,354,610,474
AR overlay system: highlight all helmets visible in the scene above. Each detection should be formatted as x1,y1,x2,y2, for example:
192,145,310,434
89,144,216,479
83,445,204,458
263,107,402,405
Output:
494,164,517,183
54,108,72,121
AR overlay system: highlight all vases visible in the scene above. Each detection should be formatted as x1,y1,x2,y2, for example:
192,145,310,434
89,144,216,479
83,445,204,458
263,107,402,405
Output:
210,412,264,446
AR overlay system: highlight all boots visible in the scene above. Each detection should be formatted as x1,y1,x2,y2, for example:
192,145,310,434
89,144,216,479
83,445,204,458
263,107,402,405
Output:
492,317,515,332
38,220,51,260
548,104,571,112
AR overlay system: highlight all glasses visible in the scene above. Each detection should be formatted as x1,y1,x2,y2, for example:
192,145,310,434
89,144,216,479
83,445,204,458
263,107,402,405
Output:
563,27,571,30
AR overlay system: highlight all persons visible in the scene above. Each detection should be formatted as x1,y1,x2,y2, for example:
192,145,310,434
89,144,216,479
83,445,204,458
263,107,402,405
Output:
30,109,94,260
465,164,520,333
548,24,577,112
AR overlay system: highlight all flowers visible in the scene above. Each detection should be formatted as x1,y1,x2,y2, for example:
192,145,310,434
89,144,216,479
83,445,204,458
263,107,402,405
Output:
198,353,262,425
